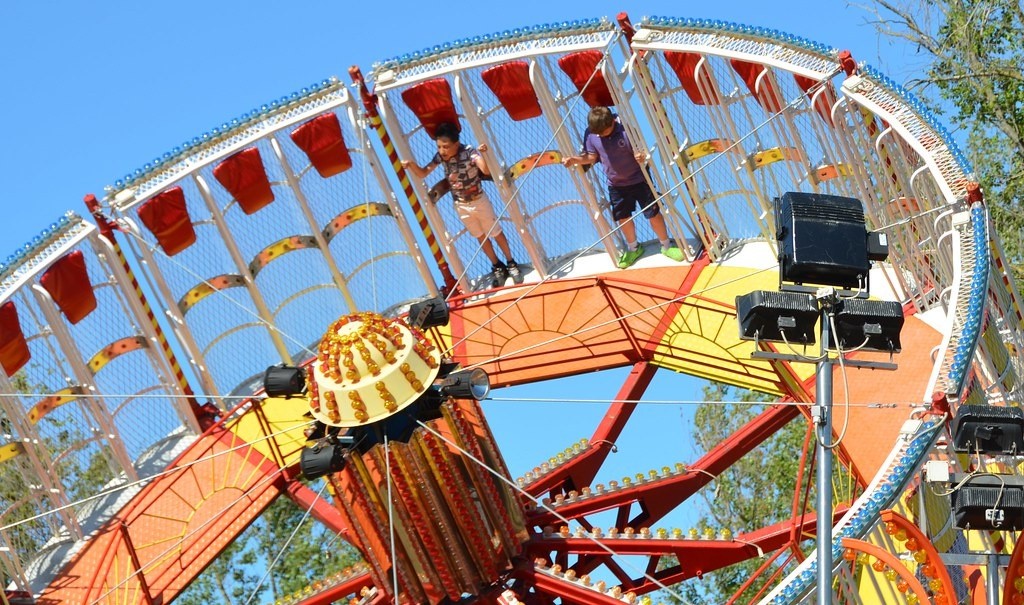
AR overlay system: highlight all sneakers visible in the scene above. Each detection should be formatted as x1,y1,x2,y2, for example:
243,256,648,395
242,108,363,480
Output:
491,266,509,288
506,258,524,283
619,242,644,269
661,245,685,262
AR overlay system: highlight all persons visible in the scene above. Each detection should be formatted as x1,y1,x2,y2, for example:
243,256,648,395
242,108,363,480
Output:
562,105,685,268
400,121,524,288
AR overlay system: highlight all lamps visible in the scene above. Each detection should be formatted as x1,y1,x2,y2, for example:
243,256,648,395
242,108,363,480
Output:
440,367,490,402
408,294,450,333
262,362,309,401
298,428,347,479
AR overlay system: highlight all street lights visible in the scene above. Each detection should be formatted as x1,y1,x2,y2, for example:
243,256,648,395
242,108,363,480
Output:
732,190,906,605
947,404,1024,605
